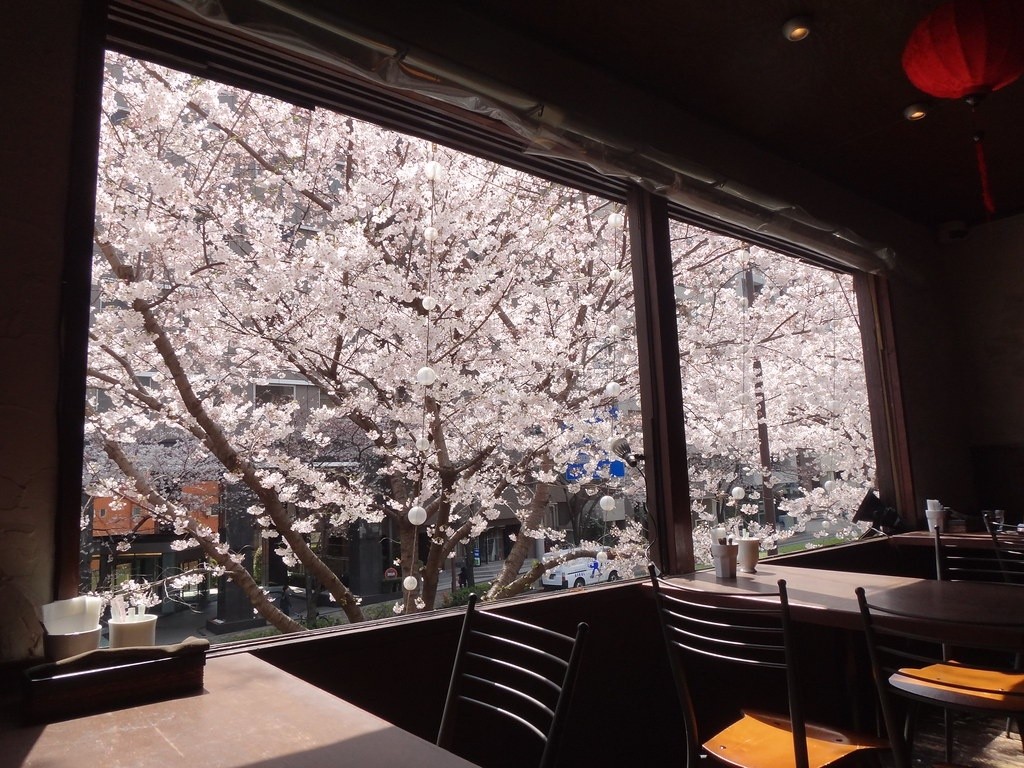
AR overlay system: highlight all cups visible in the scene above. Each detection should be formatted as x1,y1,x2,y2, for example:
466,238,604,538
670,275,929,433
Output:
925,510,946,532
736,538,760,572
711,543,738,577
43,625,102,662
982,510,1004,533
108,614,158,648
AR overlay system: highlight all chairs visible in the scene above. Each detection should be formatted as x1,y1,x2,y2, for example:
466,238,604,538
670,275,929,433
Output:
648,564,891,768
983,513,1024,737
887,525,1024,763
855,586,1024,768
435,592,590,768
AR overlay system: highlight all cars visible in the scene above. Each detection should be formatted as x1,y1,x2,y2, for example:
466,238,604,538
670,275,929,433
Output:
542,558,635,589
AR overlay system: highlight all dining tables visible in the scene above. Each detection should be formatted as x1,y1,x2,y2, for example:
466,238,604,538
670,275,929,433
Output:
638,564,1024,768
0,651,484,768
887,529,1024,584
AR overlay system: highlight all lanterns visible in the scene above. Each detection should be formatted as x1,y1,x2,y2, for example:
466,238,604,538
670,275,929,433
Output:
900,0,1024,211
